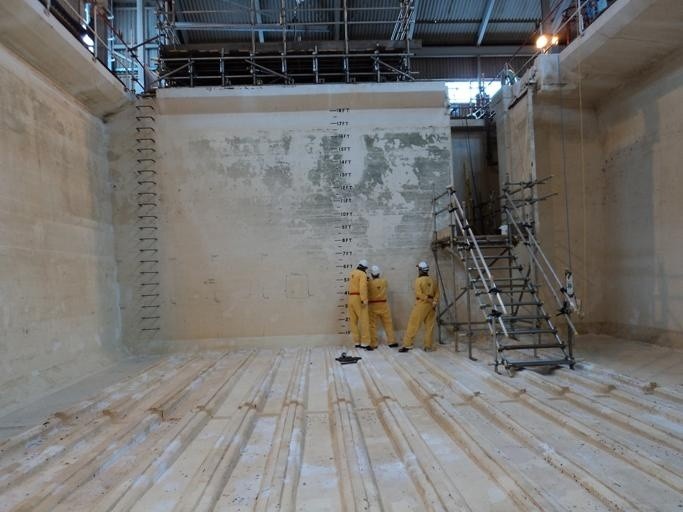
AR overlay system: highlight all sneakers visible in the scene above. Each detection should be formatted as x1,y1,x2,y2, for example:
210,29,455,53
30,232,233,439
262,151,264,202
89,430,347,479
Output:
355,345,378,350
388,343,408,352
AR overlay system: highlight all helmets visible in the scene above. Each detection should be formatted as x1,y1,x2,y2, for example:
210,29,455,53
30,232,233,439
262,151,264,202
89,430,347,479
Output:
359,259,369,268
371,266,380,276
416,262,429,272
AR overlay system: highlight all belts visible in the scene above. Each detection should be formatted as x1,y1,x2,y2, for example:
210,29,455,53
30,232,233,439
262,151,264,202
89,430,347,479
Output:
350,293,360,295
367,300,385,302
416,296,433,299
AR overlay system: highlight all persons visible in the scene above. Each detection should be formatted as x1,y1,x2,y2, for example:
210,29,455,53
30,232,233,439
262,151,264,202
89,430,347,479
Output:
368,265,398,350
346,258,373,349
395,261,441,353
